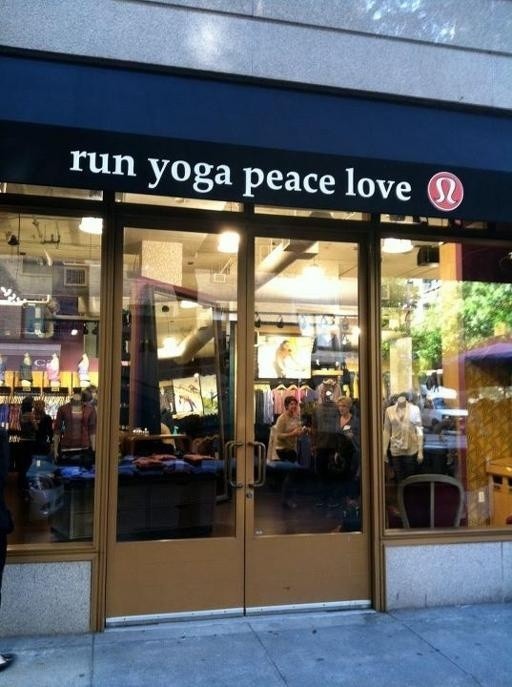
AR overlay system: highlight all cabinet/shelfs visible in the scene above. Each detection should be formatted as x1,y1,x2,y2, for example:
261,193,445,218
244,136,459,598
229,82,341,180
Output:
486,458,512,525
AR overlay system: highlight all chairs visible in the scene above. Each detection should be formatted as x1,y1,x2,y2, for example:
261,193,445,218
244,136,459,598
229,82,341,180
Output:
389,473,463,528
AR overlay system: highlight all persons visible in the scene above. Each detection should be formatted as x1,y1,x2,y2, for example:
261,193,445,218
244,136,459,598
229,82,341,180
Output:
331,395,361,507
380,395,422,526
177,394,197,411
18,396,36,487
46,351,62,386
274,395,308,509
31,399,54,462
52,393,96,469
19,351,33,390
0,357,7,386
271,339,291,379
77,352,90,385
70,384,97,415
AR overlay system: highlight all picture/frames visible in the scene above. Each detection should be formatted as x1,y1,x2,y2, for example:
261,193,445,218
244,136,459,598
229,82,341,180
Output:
63,261,89,288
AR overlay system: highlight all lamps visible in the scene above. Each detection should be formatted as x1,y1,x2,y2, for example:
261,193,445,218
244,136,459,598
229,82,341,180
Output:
254,313,285,328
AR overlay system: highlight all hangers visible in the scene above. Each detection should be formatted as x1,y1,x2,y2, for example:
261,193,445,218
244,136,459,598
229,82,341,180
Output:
275,382,313,391
